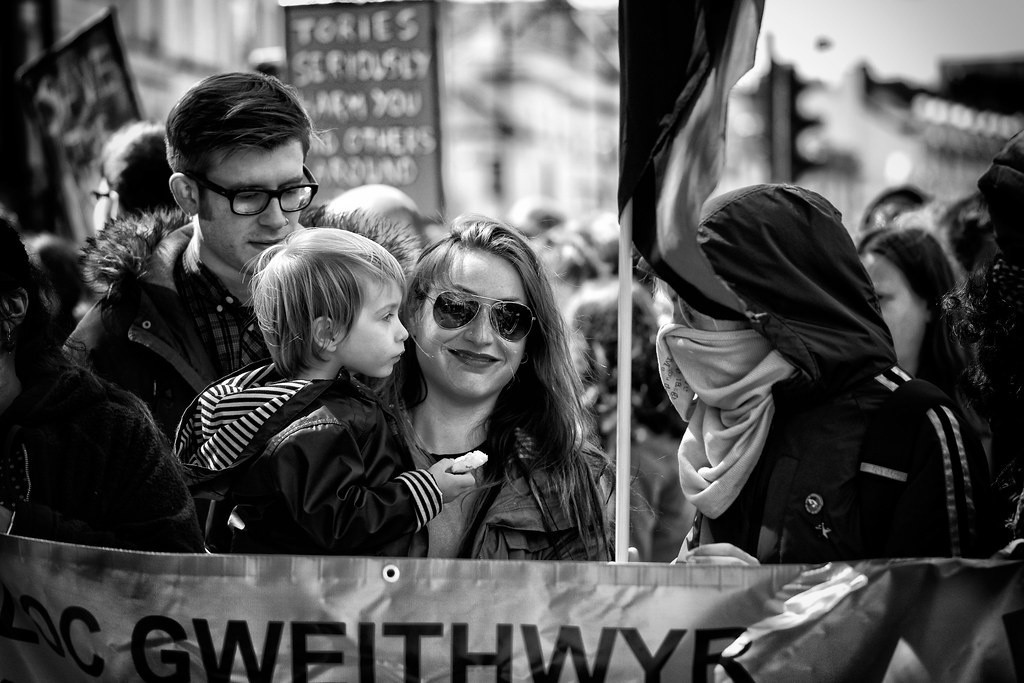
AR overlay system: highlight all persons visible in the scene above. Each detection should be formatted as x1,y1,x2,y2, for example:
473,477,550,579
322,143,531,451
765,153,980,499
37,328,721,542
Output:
166,223,481,557
366,220,618,556
77,117,1018,440
853,226,1000,472
553,275,698,560
63,67,318,462
0,219,210,559
638,182,999,557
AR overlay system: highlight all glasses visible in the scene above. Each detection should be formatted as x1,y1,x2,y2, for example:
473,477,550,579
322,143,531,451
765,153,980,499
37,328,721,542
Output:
424,289,537,342
182,163,320,217
